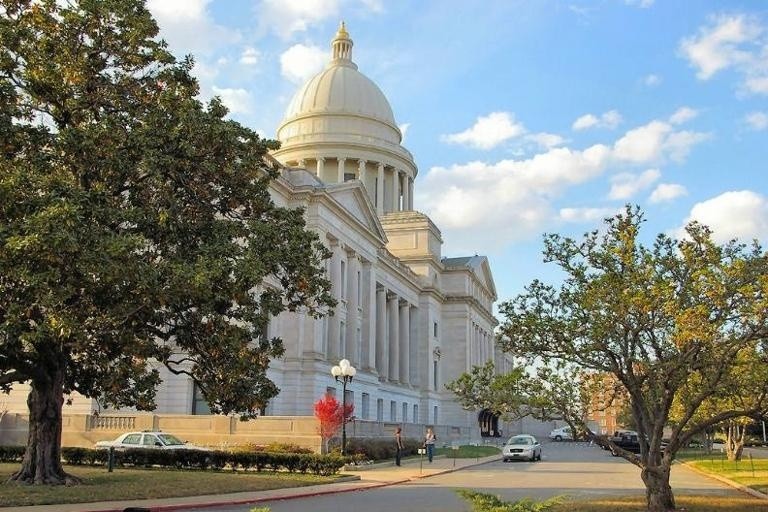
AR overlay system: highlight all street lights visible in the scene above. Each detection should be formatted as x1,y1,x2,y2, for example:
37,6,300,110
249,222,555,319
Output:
331,359,356,454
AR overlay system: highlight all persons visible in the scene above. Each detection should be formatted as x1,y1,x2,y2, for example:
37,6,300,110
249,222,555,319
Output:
393,427,405,466
425,426,437,463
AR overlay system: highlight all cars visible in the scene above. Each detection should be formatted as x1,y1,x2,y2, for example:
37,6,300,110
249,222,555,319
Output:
503,435,543,462
95,430,209,453
608,430,667,456
550,426,596,443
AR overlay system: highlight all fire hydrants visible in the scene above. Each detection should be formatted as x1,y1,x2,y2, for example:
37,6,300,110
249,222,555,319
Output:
108,446,114,472
